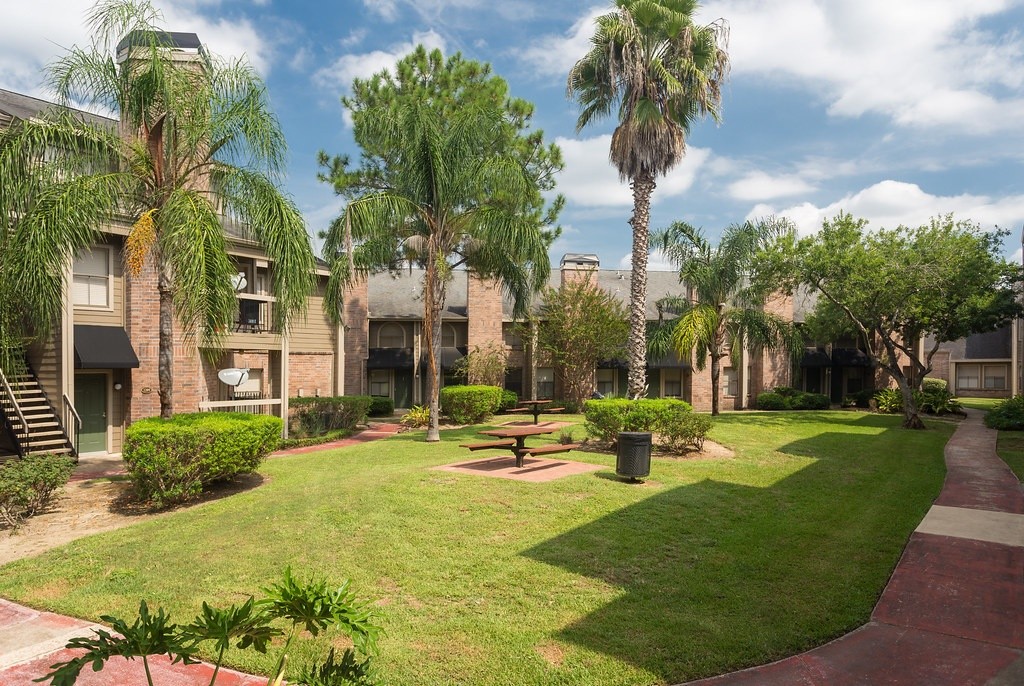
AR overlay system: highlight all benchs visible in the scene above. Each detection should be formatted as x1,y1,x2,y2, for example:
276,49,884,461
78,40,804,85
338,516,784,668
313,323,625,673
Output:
519,444,577,457
544,408,565,412
506,408,529,411
459,440,517,451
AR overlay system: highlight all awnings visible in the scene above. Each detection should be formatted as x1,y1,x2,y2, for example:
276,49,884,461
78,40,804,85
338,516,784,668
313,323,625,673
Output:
441,347,467,368
367,347,414,368
615,344,692,367
73,325,139,369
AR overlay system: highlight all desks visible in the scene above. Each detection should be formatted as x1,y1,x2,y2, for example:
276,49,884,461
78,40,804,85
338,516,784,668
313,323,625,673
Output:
518,400,553,425
477,427,561,468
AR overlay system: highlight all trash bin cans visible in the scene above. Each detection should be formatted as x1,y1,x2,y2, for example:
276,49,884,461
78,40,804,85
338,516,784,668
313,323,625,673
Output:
615,432,653,477
